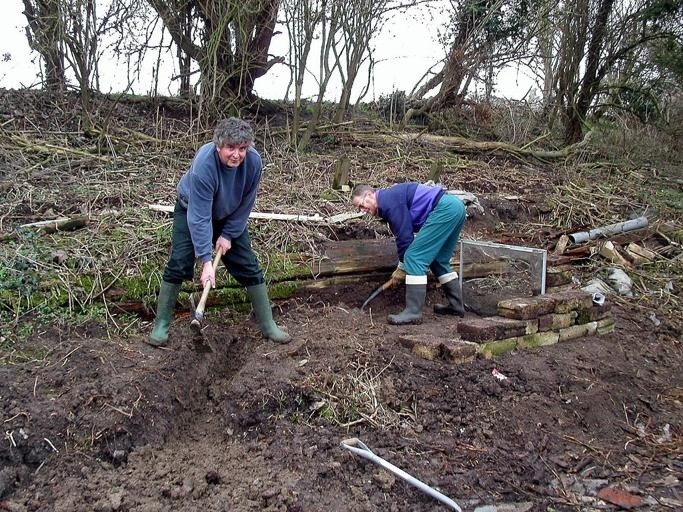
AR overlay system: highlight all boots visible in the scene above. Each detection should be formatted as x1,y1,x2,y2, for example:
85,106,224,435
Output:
434,272,464,318
150,280,182,345
388,275,427,324
247,282,291,343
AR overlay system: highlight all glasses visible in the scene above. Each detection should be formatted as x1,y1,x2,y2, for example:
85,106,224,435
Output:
357,192,367,213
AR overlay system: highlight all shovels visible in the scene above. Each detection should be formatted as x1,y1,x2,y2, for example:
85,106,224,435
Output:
339,436,536,511
149,204,366,224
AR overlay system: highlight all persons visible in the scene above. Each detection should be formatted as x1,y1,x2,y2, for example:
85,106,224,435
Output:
147,115,293,346
349,181,468,326
